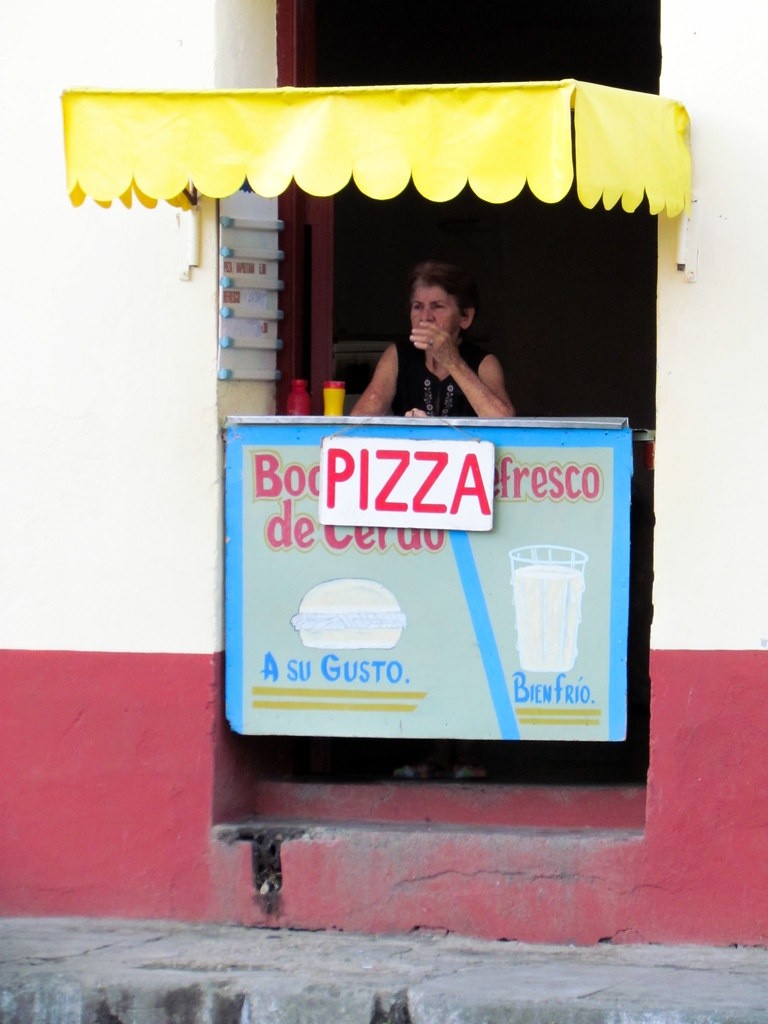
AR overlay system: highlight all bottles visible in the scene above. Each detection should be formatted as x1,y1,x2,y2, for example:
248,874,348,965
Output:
286,379,311,415
323,380,345,416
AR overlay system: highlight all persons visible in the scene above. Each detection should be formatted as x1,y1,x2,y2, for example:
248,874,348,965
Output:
348,263,514,782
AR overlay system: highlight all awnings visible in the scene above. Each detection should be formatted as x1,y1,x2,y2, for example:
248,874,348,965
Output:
58,73,697,223
